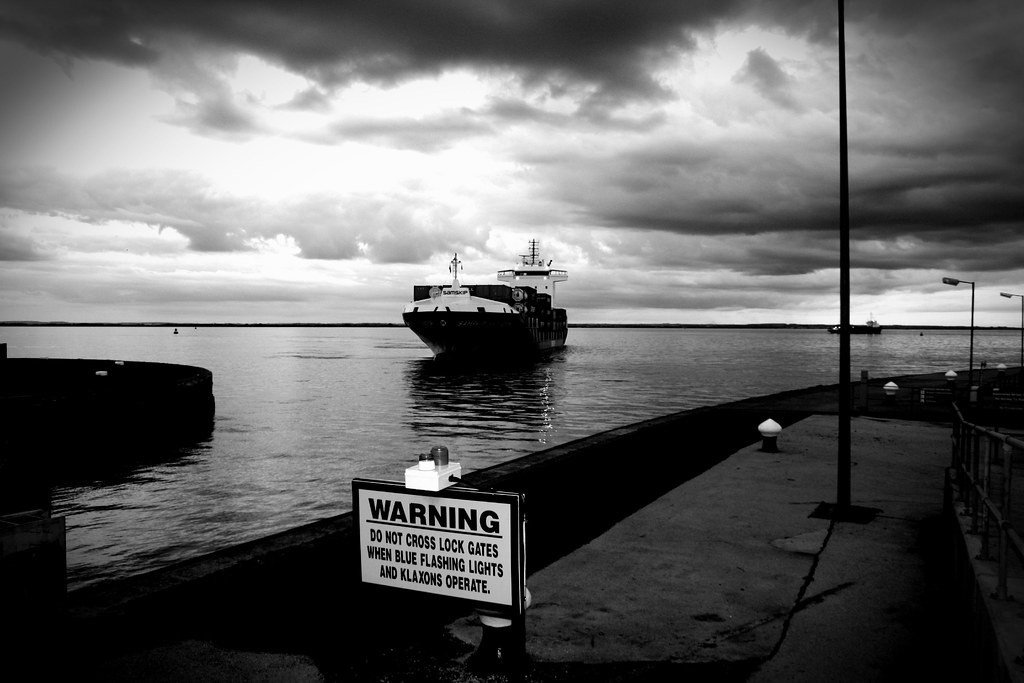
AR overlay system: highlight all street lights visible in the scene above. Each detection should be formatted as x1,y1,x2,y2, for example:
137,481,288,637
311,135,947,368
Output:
999,291,1023,372
942,276,975,388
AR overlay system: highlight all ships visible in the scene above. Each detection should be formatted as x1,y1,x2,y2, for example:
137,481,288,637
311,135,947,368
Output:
401,239,570,366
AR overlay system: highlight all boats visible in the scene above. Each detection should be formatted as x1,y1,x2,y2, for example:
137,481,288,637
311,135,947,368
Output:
827,311,881,335
